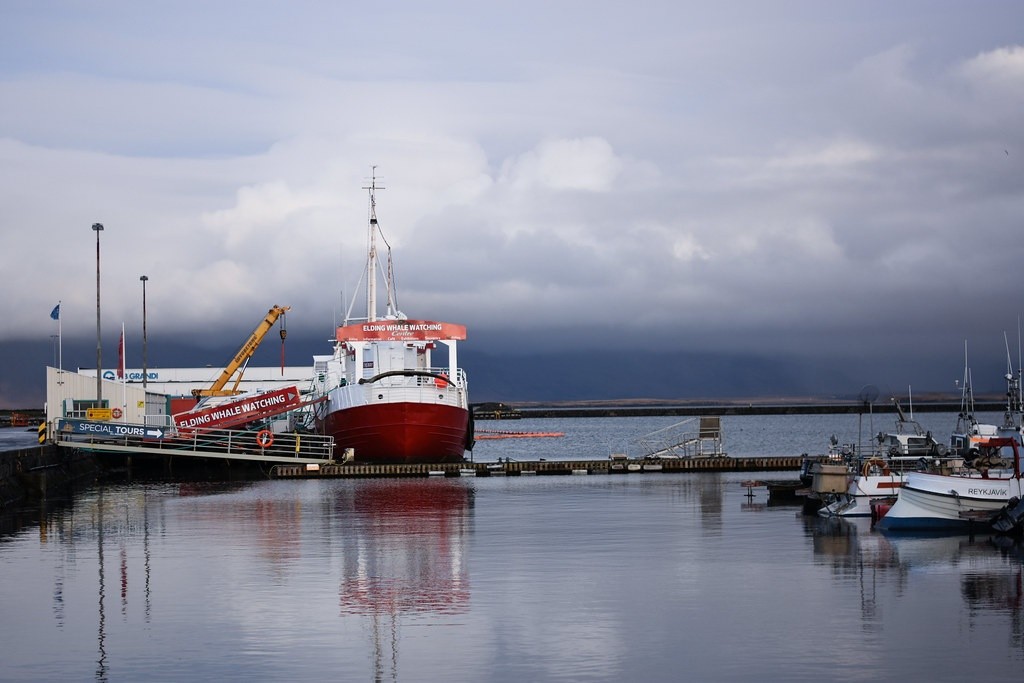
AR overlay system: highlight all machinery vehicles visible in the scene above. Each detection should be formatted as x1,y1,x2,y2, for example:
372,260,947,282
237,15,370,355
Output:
191,304,292,402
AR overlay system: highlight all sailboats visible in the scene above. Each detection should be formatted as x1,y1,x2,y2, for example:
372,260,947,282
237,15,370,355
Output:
875,315,1019,529
312,164,470,464
818,340,1018,522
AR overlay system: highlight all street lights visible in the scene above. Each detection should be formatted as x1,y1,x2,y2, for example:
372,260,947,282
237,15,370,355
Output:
139,275,148,388
92,221,105,408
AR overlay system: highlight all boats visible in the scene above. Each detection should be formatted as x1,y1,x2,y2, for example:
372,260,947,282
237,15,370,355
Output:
196,393,315,436
758,477,804,501
998,411,1017,430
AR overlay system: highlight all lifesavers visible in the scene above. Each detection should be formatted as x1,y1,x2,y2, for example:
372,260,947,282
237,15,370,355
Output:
863,460,890,476
257,430,274,447
112,407,122,419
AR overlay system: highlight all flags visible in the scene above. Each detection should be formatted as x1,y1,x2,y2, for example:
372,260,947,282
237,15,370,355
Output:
50,304,59,320
117,331,123,379
